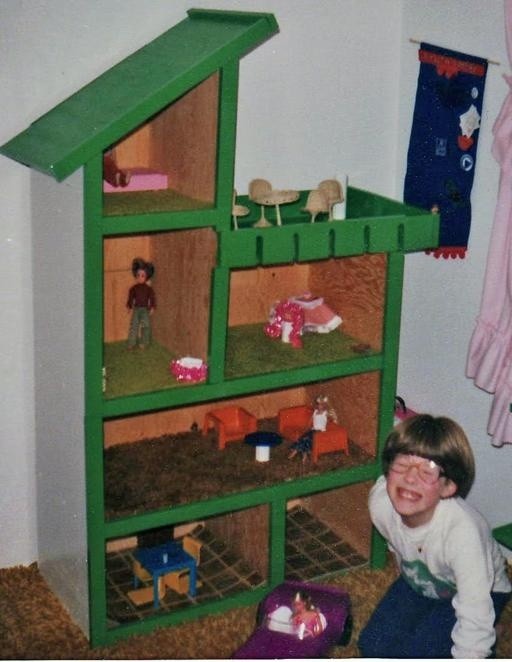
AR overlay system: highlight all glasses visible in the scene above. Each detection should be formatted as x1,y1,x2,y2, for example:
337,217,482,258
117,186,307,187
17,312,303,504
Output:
388,454,449,484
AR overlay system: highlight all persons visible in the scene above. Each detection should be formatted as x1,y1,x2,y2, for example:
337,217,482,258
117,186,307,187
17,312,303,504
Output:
356,413,512,659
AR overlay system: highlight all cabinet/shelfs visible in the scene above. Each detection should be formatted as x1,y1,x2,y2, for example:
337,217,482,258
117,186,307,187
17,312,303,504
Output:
1,8,441,650
